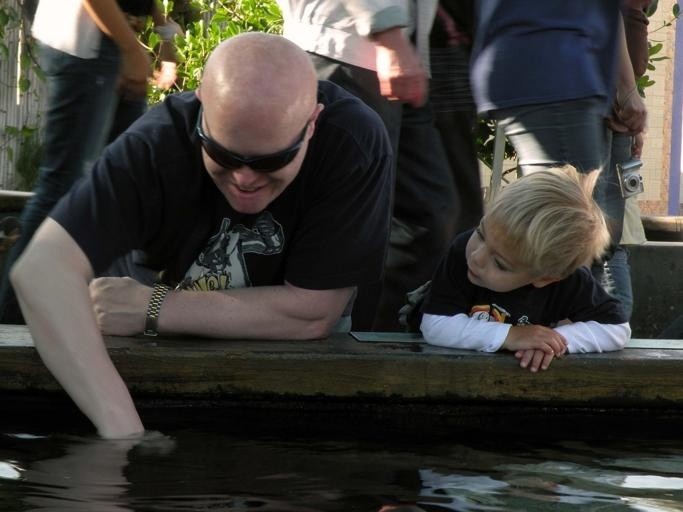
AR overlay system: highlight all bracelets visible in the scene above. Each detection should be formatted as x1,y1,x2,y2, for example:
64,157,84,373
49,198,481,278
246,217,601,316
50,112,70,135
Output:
157,22,182,41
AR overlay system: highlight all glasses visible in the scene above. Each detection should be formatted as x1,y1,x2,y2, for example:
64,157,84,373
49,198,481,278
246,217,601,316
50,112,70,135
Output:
193,104,309,174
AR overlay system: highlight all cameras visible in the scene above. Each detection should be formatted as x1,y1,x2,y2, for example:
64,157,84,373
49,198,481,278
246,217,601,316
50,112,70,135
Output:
615,160,645,199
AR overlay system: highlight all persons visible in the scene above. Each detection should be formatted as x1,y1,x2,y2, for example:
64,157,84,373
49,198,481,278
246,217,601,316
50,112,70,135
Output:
277,1,459,331
107,2,185,142
1,0,152,326
7,29,395,454
399,159,632,374
410,3,654,320
